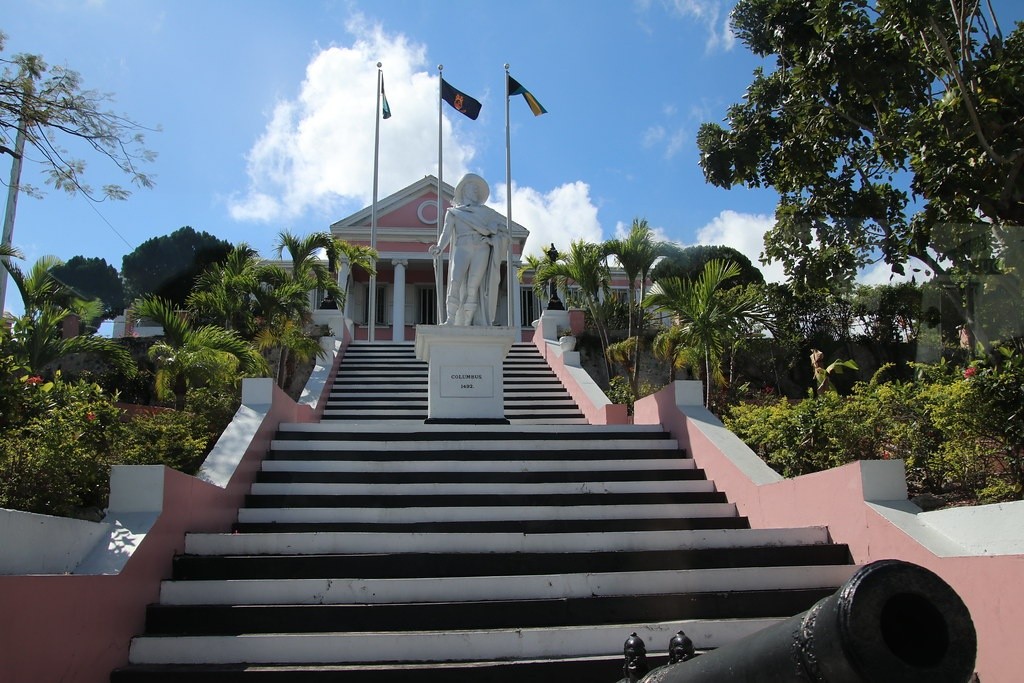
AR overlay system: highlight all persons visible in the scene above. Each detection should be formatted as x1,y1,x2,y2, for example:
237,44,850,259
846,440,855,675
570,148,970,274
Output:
428,174,510,325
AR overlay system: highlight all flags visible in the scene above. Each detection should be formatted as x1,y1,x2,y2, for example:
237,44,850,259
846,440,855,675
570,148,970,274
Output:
509,76,549,117
442,78,482,121
381,72,391,119
